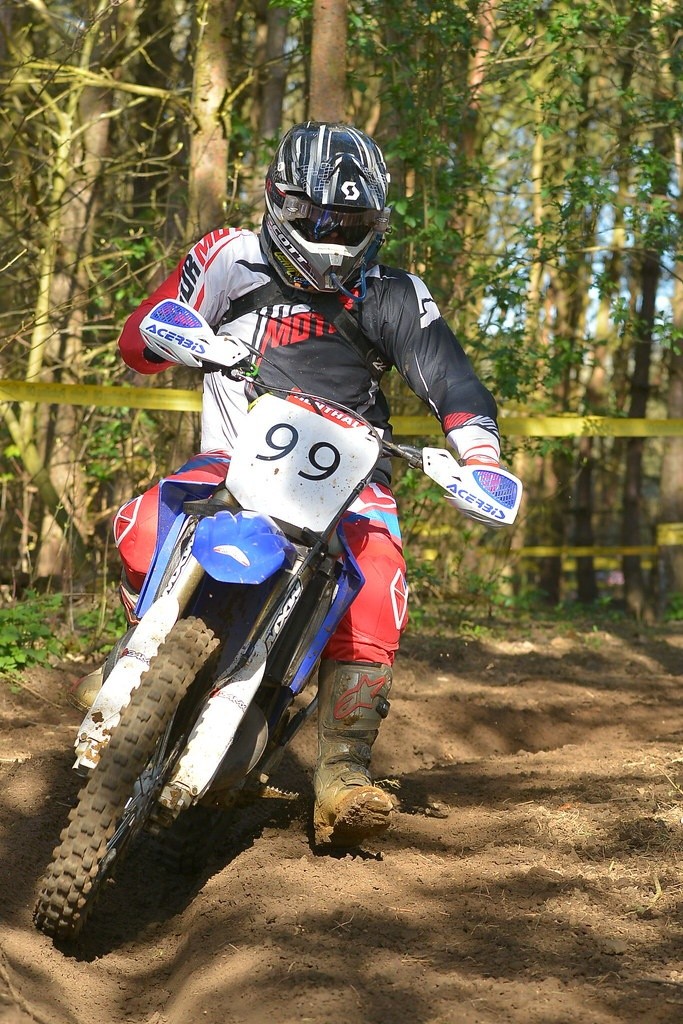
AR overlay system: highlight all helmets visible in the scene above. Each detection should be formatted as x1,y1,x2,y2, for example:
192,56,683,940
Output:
259,123,392,293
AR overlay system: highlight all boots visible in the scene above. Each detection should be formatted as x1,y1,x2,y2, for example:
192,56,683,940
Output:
66,568,142,714
313,661,393,850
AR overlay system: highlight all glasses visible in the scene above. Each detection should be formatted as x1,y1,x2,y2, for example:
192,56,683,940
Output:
265,167,391,247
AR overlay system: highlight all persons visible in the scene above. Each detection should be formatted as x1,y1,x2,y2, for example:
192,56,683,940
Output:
68,115,501,854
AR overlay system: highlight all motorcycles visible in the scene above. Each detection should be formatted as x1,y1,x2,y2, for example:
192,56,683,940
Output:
30,296,525,944
